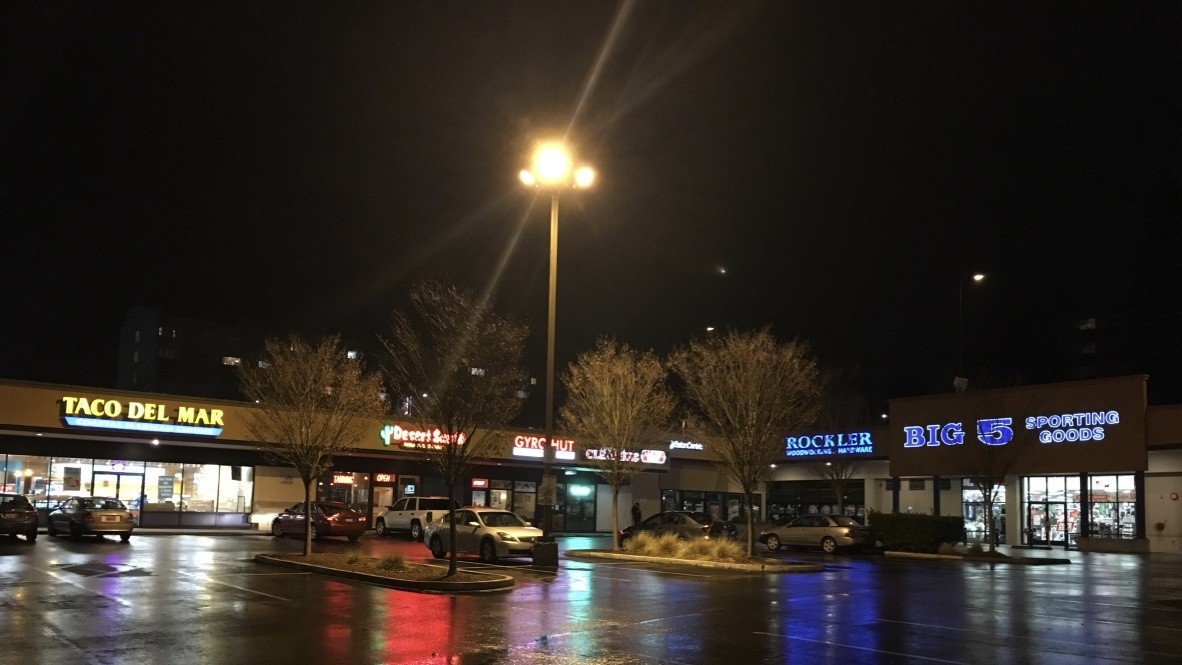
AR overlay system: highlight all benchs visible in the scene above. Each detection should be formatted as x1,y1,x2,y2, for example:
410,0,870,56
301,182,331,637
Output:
187,498,215,512
26,493,70,508
141,501,177,512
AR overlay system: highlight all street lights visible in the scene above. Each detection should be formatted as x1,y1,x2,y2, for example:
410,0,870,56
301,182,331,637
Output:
519,140,596,568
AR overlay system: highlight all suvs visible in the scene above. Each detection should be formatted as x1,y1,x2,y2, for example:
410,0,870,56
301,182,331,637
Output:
375,496,461,539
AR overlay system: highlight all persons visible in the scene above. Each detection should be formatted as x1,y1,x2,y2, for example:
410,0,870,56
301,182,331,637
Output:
632,502,641,526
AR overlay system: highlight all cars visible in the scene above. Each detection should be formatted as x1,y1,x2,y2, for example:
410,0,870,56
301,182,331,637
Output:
0,492,39,542
47,496,134,543
425,508,545,562
280,498,366,543
759,515,876,554
617,510,738,547
